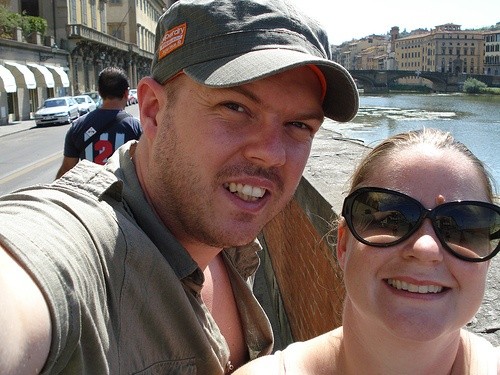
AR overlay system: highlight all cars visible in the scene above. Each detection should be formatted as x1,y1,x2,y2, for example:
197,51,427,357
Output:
35,96,80,128
126,89,139,107
83,91,104,109
75,95,97,116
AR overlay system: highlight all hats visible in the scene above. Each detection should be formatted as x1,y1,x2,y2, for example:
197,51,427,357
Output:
151,0,359,120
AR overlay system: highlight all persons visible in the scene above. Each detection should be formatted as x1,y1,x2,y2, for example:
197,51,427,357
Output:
0,0,358,375
54,66,143,180
229,129,500,375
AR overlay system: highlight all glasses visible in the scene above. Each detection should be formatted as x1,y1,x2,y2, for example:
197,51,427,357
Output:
342,187,500,263
